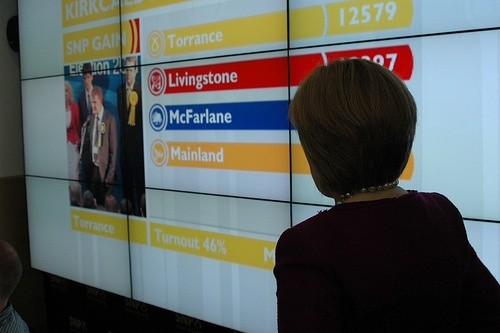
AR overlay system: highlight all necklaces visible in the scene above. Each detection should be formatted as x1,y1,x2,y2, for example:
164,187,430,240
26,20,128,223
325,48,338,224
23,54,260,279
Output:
334,178,400,203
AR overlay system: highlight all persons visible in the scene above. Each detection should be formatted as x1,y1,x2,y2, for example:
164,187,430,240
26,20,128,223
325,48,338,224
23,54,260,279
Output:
81,188,99,210
116,55,145,217
139,192,146,217
272,56,500,333
0,234,29,333
68,180,84,206
117,199,134,216
78,85,119,208
104,194,119,214
63,79,83,190
75,61,103,136
